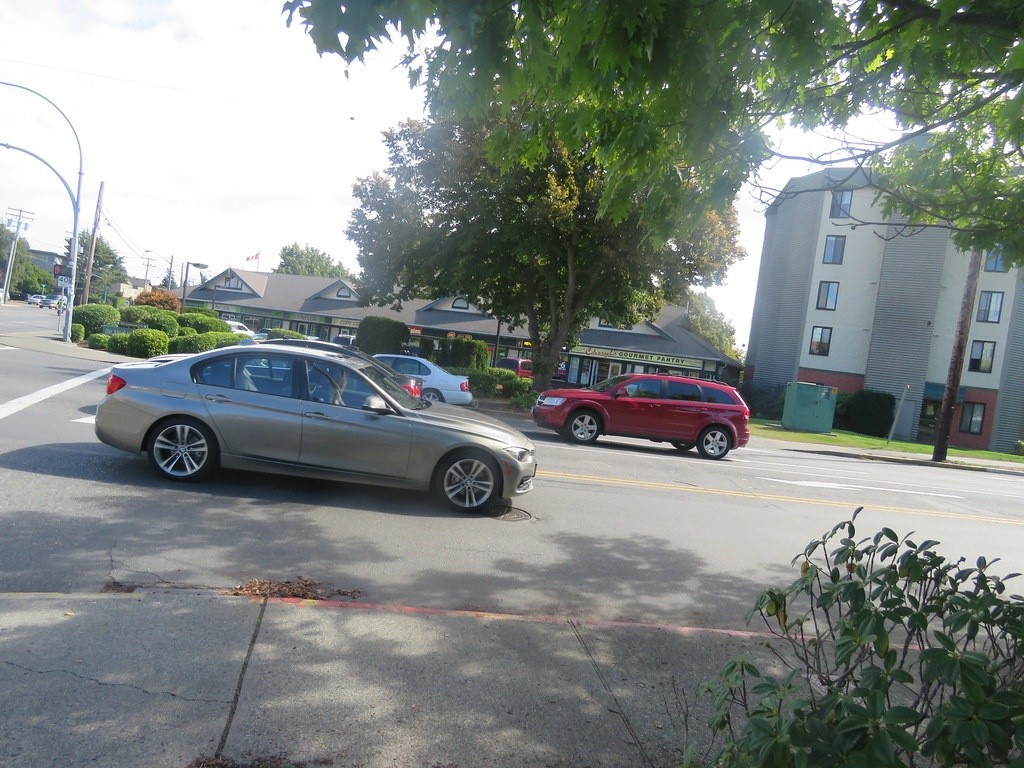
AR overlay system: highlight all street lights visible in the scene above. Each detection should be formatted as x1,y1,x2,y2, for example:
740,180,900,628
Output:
180,262,209,314
212,284,221,311
143,249,174,292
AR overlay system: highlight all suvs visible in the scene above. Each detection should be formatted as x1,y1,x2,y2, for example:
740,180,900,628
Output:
38,294,67,309
529,373,751,460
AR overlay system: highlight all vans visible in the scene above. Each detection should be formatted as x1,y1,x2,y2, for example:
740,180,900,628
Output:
333,334,357,347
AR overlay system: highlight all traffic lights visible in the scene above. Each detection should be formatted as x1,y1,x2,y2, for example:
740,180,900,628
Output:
54,263,62,275
63,237,76,262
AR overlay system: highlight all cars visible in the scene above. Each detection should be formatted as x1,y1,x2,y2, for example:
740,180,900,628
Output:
27,294,47,305
225,320,256,337
94,344,538,515
371,354,473,405
20,293,32,301
554,361,567,379
256,328,269,339
496,357,535,379
0,289,11,303
146,337,423,400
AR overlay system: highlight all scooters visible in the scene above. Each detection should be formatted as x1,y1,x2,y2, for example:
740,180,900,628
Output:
56,303,66,316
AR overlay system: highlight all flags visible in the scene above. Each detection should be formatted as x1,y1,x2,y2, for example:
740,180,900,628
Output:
246,253,260,261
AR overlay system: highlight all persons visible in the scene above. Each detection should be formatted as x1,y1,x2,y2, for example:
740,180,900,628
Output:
321,369,347,405
57,299,65,308
